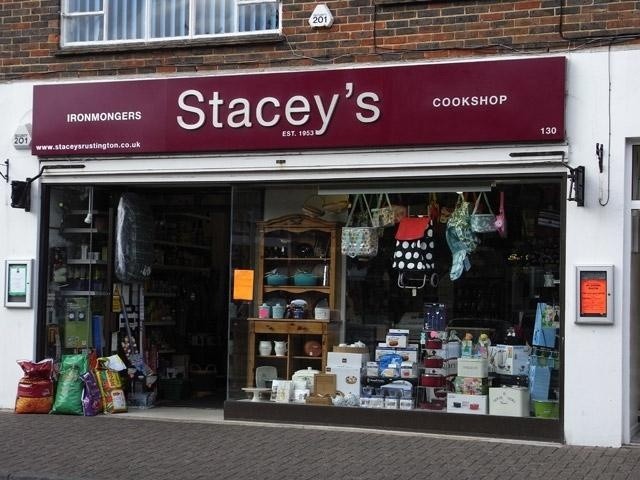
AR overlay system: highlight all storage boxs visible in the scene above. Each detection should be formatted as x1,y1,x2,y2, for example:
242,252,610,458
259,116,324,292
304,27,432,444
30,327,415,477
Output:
270,329,559,420
159,376,193,400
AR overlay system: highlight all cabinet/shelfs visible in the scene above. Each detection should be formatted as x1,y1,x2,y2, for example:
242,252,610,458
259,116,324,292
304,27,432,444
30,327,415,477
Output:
55,210,217,381
254,213,346,320
247,318,343,396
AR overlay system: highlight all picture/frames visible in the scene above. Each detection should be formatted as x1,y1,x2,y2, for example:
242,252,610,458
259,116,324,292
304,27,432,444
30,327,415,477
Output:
5,259,32,308
574,267,614,325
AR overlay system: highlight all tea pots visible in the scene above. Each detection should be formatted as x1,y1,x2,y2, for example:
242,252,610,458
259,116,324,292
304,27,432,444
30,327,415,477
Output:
274,341,289,356
345,392,359,407
331,395,347,407
498,350,507,369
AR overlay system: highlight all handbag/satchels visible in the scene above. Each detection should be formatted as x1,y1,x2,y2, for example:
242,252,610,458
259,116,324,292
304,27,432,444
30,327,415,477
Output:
440,207,455,224
496,214,508,239
371,207,395,228
471,214,496,233
382,204,407,223
446,194,482,281
341,227,379,257
427,200,440,222
353,212,385,238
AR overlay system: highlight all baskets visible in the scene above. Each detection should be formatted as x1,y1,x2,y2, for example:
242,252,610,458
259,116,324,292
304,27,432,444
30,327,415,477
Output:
301,195,325,219
190,364,219,390
127,381,155,407
158,379,185,401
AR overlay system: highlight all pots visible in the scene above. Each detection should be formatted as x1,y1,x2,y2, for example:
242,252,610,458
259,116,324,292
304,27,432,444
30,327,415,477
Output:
420,370,458,387
426,336,458,348
267,273,290,285
423,352,458,369
346,375,357,385
420,398,445,411
294,272,318,286
434,374,458,398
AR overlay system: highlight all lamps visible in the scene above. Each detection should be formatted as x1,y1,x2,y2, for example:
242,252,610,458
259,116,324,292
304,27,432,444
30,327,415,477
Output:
317,180,496,195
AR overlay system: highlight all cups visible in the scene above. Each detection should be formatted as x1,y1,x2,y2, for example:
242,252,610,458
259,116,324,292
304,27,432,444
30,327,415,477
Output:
258,303,270,319
273,304,284,318
